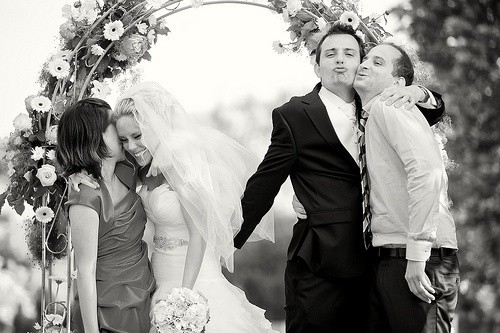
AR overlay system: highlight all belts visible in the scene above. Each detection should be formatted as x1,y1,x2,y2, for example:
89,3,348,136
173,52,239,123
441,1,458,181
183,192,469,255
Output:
378,246,454,258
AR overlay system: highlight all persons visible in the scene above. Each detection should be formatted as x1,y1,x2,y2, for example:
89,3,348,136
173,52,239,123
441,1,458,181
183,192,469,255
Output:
55,97,157,333
219,24,446,333
68,89,275,333
292,40,460,333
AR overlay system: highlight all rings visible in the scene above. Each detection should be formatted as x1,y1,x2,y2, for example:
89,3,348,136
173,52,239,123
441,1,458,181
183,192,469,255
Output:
80,178,83,183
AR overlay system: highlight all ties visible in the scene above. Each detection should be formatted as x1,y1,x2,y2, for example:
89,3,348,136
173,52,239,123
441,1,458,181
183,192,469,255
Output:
339,103,357,129
358,110,372,250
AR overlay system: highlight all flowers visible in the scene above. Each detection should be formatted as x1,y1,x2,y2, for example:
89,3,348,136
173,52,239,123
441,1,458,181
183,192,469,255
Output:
149,286,211,333
0,1,457,333
